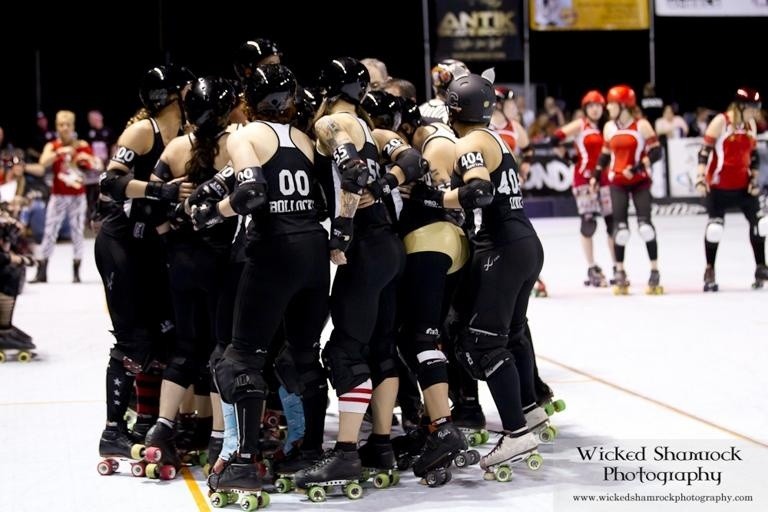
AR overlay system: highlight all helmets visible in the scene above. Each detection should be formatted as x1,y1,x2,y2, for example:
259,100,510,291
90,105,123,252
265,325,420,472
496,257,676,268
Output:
398,96,422,121
184,76,236,128
445,74,495,125
581,91,606,106
495,86,516,102
319,58,368,102
139,64,197,116
433,60,471,94
0,218,23,242
242,37,284,68
244,62,296,115
75,146,94,171
737,87,760,104
360,91,398,129
606,85,636,108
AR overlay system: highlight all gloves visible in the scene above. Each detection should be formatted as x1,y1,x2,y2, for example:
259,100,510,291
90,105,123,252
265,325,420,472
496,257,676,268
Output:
592,168,602,182
193,198,225,230
749,175,759,188
168,200,190,226
695,173,707,187
410,179,444,208
368,172,399,200
145,181,182,202
329,216,354,252
629,161,644,174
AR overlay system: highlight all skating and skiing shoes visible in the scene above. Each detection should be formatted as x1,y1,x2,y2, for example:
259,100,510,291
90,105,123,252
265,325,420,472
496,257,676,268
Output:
411,422,481,487
537,382,566,415
585,266,608,285
532,280,548,298
295,449,364,502
359,442,399,488
206,463,270,510
178,431,207,467
481,428,542,483
266,450,323,492
646,270,662,295
611,266,630,286
0,330,35,364
752,267,766,291
614,271,631,295
704,270,718,292
452,405,490,447
132,422,182,480
522,402,557,442
97,431,134,475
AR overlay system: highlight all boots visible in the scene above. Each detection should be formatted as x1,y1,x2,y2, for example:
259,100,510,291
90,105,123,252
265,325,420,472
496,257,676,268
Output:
72,259,82,283
27,258,50,284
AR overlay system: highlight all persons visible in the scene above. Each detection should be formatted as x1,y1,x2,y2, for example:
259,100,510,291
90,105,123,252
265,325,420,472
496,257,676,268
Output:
400,73,558,483
417,57,470,127
292,56,403,503
355,89,480,488
79,110,115,220
189,61,333,512
696,88,768,292
654,105,690,139
383,82,415,103
372,96,490,448
95,63,197,486
0,209,37,362
688,107,711,137
360,57,393,93
128,74,248,481
1,150,13,169
589,84,663,296
232,37,278,73
29,111,50,161
545,97,565,127
3,161,47,218
641,83,663,126
551,90,618,287
29,111,95,284
487,86,533,185
